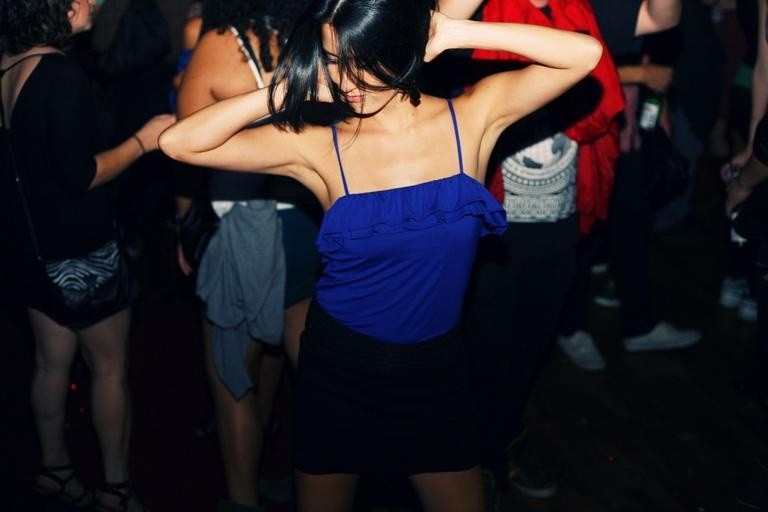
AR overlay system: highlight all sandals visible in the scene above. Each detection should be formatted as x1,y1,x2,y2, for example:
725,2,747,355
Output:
95,475,145,512
35,464,92,511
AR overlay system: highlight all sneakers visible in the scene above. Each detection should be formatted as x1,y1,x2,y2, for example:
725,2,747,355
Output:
624,324,701,352
556,332,607,371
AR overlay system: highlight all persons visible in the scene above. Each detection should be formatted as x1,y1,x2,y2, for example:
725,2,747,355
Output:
1,0,768,512
